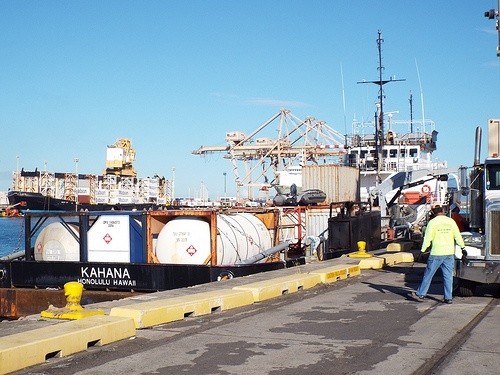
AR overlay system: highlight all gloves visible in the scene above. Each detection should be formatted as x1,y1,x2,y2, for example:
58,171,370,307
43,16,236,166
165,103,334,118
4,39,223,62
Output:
461,248,468,258
419,251,425,259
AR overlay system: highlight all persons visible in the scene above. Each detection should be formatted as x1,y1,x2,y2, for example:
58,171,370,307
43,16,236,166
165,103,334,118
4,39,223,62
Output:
415,201,467,304
450,203,465,232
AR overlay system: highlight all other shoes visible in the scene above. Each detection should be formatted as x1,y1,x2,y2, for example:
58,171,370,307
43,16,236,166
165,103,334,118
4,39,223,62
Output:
407,292,425,302
441,298,453,304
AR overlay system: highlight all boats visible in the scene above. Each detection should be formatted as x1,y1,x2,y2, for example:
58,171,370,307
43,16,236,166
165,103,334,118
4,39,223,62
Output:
1,27,447,323
